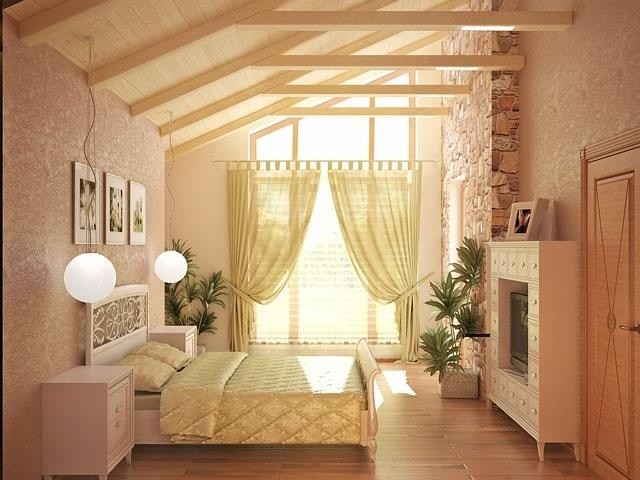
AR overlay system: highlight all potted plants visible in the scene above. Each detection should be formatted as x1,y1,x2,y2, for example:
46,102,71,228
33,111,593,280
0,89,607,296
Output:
417,236,486,400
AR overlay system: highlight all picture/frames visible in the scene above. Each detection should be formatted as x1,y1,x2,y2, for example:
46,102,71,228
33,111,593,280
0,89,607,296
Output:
71,161,147,247
505,198,550,241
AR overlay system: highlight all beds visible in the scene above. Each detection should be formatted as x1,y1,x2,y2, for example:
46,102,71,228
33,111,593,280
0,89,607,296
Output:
85,282,382,464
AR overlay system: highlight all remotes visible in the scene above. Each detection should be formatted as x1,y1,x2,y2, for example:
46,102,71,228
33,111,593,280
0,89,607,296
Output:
506,368,525,376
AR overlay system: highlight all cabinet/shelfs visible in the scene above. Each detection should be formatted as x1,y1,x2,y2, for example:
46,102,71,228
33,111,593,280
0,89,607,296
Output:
484,240,584,462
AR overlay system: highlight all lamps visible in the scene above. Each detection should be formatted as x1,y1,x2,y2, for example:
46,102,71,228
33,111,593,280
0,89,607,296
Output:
154,112,188,285
63,35,117,304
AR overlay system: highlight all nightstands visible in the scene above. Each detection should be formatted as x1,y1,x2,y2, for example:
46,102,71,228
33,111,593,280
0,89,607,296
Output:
149,325,198,358
40,365,136,480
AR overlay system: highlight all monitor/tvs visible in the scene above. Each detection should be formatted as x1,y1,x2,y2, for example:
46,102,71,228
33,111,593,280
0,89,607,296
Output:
511,292,528,374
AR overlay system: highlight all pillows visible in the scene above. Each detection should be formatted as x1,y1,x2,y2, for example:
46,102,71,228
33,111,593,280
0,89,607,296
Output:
111,341,195,393
